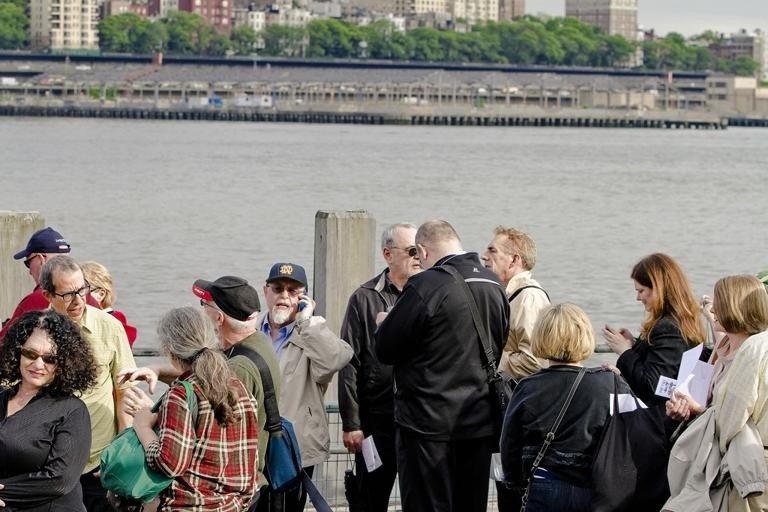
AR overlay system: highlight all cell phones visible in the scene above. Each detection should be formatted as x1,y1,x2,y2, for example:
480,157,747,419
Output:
606,324,619,335
299,298,305,312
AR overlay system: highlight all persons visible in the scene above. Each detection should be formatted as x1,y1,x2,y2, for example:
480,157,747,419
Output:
81,261,137,348
0,228,101,344
339,222,423,512
123,307,259,512
0,310,98,512
700,295,724,342
480,225,552,512
116,276,281,512
601,253,713,512
39,255,137,512
665,275,768,512
500,303,630,512
372,219,510,512
257,263,355,512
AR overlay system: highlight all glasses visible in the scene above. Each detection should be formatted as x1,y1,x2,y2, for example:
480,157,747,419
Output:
52,278,91,303
390,246,418,258
20,347,60,364
198,298,220,313
20,254,48,268
267,283,305,297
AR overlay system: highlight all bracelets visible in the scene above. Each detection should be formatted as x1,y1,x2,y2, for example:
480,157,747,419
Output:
295,315,311,325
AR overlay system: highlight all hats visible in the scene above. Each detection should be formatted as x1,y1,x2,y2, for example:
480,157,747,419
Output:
265,262,308,286
189,275,262,322
11,226,73,263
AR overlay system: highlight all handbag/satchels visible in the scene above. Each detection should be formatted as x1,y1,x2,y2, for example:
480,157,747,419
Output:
265,413,306,500
590,367,640,512
97,381,200,504
489,366,520,435
